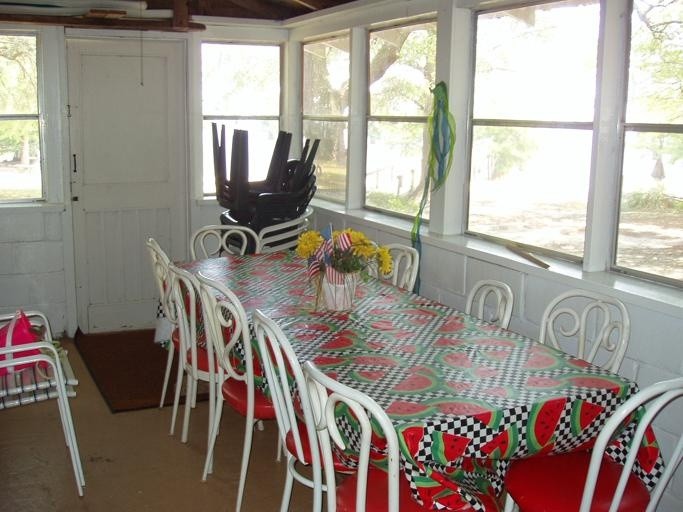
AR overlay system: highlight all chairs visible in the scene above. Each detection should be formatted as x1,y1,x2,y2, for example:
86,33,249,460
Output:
1,312,87,497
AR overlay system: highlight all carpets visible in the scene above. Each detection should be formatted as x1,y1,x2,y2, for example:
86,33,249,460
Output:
72,328,210,413
